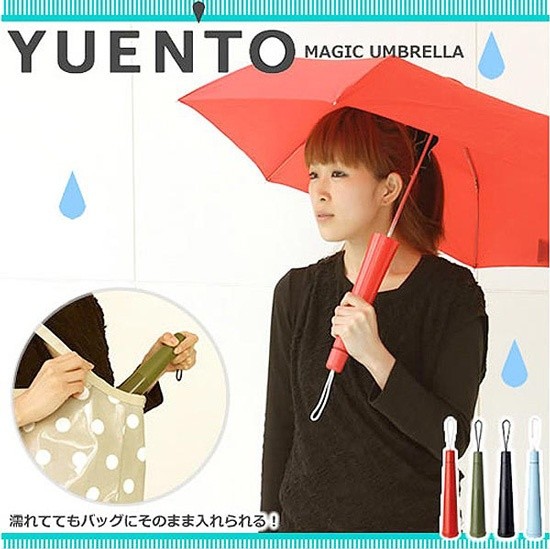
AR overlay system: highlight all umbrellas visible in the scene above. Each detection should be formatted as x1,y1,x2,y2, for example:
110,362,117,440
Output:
178,56,549,422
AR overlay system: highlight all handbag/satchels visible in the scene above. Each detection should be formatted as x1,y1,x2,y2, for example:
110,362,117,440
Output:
13,324,146,503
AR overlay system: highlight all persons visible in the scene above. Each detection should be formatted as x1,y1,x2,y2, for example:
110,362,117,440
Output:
13,295,199,428
260,107,489,533
